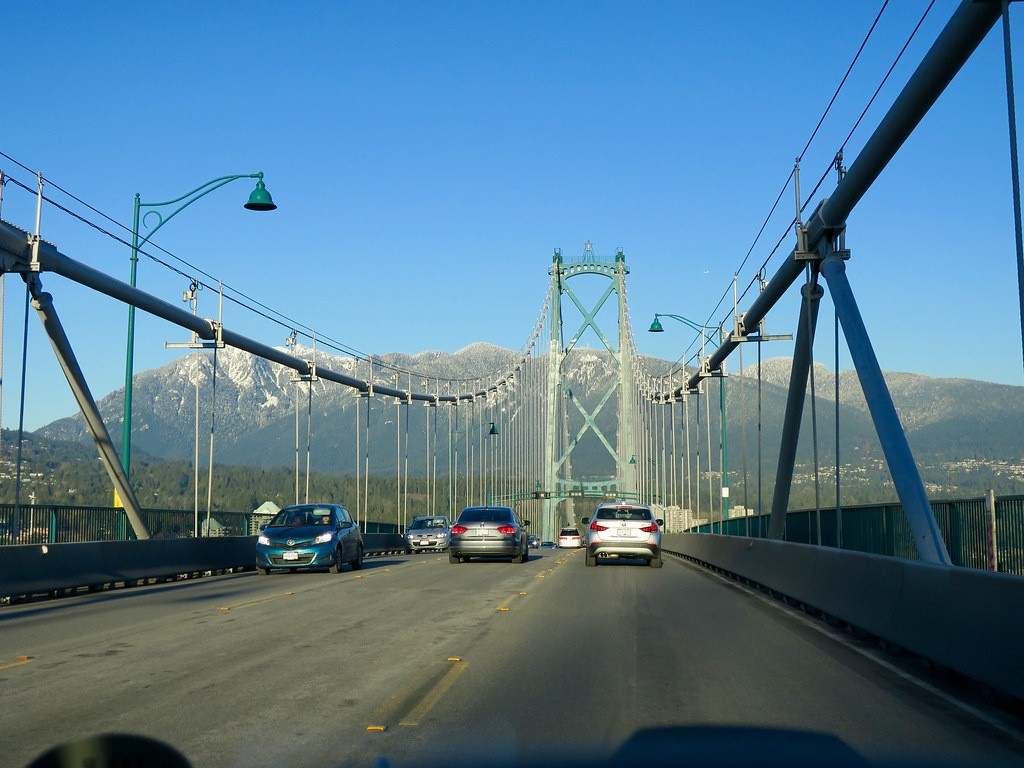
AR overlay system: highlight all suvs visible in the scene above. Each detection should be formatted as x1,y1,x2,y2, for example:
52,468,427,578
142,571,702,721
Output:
582,502,664,568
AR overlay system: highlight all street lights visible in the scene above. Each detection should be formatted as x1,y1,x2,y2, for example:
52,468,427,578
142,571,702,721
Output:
451,421,499,519
648,313,729,519
120,171,277,538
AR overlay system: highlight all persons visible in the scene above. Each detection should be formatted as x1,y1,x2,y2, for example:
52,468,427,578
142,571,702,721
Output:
315,515,331,524
437,522,444,527
294,517,304,524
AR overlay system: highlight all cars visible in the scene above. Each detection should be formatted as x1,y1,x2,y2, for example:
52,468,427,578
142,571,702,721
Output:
449,504,531,563
405,516,452,554
527,535,540,549
256,503,363,575
540,542,557,548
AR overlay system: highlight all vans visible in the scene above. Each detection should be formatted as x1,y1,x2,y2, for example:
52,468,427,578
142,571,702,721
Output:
558,528,582,549
